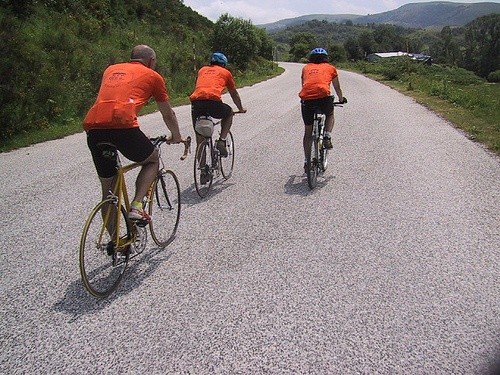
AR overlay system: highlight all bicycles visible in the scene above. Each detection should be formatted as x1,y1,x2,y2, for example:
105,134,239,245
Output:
300,97,348,190
79,134,191,299
194,110,247,198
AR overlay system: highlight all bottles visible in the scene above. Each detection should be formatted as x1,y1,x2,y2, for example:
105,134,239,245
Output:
214,139,218,151
319,125,324,139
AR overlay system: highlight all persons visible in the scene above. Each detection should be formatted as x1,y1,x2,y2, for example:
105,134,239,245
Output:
82,45,182,255
298,48,348,173
189,53,247,185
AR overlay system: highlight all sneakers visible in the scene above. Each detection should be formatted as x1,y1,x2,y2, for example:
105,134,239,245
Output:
128,207,151,223
107,240,135,256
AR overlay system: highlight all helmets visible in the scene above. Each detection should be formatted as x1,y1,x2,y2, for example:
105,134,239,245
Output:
210,53,227,66
311,48,328,56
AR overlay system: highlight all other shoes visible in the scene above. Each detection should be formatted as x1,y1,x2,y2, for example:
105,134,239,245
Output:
304,162,312,173
200,173,214,185
323,136,333,149
217,137,228,157
